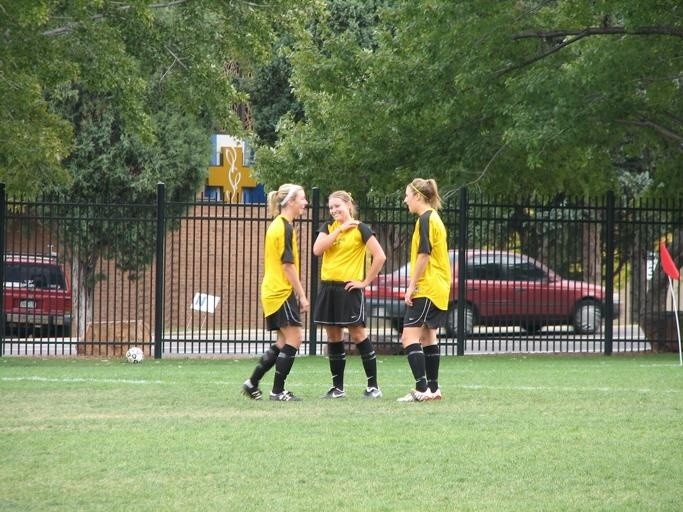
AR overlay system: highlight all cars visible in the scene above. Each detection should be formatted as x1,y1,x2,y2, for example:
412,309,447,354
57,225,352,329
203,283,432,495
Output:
365,248,621,338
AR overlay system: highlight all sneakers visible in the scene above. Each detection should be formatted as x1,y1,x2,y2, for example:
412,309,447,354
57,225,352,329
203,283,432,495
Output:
269,389,302,402
319,386,345,399
395,388,432,402
430,388,441,400
359,386,382,399
241,379,262,401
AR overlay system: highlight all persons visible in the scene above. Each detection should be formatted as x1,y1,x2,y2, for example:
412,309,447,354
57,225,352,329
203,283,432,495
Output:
394,177,454,402
236,183,310,402
312,190,388,399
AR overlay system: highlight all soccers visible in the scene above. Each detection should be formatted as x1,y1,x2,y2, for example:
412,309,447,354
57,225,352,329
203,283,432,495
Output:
126,347,143,364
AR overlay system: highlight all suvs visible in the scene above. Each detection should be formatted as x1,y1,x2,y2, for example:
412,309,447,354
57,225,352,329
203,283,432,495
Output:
4,252,82,339
